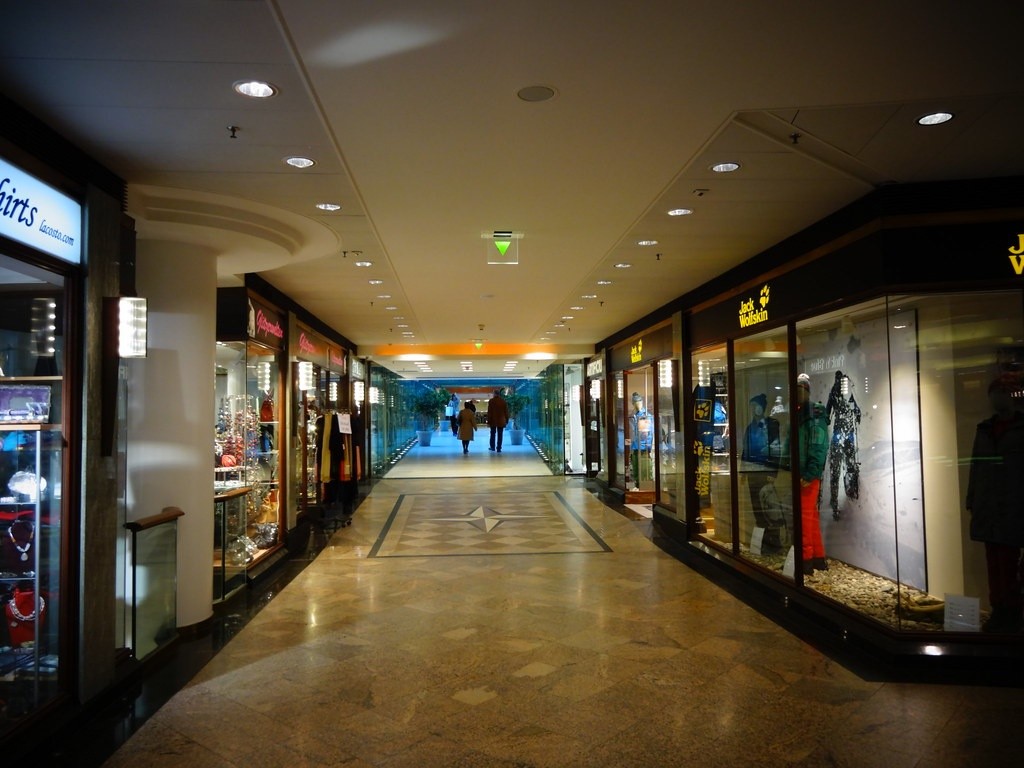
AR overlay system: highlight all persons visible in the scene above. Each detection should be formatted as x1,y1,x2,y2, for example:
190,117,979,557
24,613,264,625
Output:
629,393,653,490
698,398,728,450
307,400,317,411
739,394,780,553
469,399,477,414
449,394,459,437
456,402,477,453
487,390,509,451
781,374,828,575
0,522,35,572
967,370,1024,629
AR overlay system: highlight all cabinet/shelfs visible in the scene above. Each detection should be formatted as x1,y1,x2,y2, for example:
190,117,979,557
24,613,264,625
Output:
0,421,62,728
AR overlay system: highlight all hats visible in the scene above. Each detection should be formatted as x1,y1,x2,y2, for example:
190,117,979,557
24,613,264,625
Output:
631,392,641,404
749,393,767,412
987,374,1020,404
797,373,811,392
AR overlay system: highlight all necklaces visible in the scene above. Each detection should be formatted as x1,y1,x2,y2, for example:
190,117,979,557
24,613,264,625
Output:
9,596,45,621
8,521,34,560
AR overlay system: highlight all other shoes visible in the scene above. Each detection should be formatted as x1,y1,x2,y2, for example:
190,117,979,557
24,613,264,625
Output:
488,448,494,450
497,450,502,452
813,556,829,570
464,449,469,454
453,433,456,436
629,487,639,492
803,559,814,575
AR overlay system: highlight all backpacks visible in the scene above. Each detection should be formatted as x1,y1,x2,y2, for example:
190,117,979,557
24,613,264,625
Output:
759,482,788,545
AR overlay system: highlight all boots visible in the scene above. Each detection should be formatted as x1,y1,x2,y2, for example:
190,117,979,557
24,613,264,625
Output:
983,604,1005,633
1002,604,1023,632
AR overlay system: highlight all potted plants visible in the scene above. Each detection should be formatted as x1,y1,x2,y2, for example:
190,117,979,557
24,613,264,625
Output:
411,388,450,446
500,387,530,445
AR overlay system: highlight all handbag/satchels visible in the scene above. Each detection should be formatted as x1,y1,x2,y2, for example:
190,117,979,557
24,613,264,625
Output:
446,403,454,417
456,419,461,427
